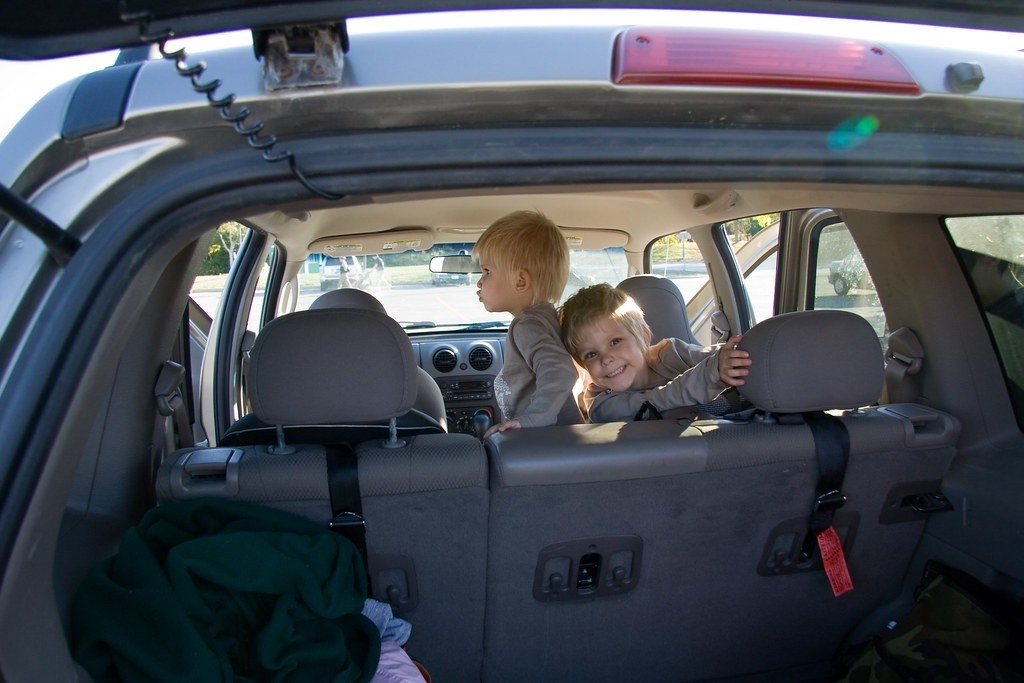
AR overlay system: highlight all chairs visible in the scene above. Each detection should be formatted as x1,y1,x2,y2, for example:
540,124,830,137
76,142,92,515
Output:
308,289,451,435
158,307,494,682
615,273,705,350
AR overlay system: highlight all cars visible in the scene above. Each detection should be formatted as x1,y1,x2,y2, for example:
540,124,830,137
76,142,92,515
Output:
827,249,877,297
319,256,364,291
430,272,472,286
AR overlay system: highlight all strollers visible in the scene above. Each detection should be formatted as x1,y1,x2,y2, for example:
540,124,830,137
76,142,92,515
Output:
352,268,372,291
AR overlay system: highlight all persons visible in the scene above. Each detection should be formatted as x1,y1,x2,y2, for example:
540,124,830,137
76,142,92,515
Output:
556,282,752,423
471,209,587,439
373,255,392,290
337,257,352,290
352,274,363,287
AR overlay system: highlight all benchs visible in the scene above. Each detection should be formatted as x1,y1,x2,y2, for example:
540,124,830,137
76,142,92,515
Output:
480,308,963,683
